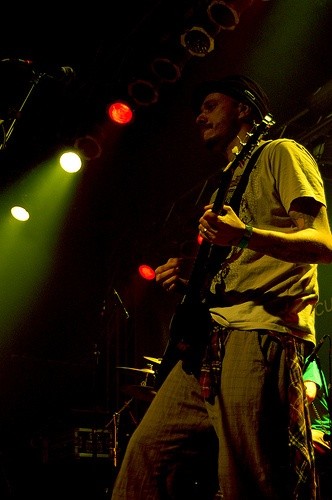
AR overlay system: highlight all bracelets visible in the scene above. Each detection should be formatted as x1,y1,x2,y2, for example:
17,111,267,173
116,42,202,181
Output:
237,223,253,249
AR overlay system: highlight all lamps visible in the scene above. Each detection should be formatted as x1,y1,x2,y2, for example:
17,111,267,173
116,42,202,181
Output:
74,0,243,162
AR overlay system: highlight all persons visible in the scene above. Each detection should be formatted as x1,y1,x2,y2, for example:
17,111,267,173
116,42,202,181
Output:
301,350,332,470
110,73,332,500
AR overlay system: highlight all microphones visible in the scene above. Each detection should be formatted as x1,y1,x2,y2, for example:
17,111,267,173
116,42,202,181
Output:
14,58,73,77
113,288,129,319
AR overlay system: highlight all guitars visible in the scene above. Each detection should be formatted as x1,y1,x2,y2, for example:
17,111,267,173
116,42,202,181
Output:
151,111,277,389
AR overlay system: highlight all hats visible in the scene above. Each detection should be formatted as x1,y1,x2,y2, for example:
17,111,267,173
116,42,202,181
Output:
209,73,272,118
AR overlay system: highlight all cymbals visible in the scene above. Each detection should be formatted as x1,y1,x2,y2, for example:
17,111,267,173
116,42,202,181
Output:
119,384,158,402
115,366,156,375
142,355,163,365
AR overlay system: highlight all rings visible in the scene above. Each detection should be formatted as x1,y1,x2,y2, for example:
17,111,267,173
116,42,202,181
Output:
201,228,207,234
